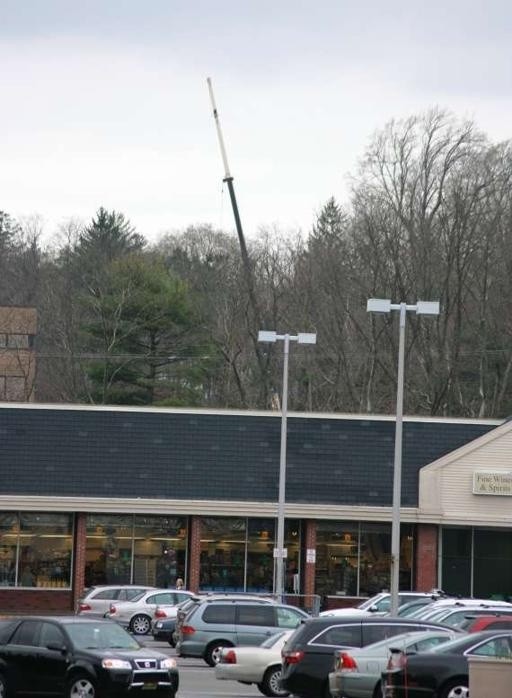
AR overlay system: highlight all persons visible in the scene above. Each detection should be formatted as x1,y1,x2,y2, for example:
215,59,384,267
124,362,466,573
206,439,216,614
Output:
50,566,66,582
175,577,185,591
20,565,37,587
367,574,382,597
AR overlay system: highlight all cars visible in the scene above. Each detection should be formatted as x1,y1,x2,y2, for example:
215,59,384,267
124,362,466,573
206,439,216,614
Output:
74,585,311,666
0,615,178,698
215,590,511,697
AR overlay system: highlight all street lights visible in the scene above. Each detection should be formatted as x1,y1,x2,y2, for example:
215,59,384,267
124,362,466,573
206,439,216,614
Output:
367,298,439,617
257,330,316,606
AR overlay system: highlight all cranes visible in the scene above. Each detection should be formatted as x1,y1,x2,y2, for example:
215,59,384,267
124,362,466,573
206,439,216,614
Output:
206,78,264,328
